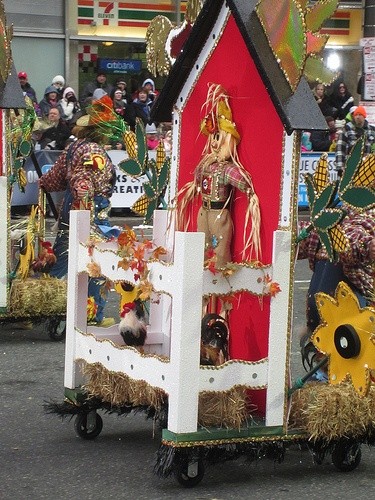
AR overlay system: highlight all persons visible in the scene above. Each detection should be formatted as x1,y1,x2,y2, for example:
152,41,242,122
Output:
310,82,375,154
39,96,117,328
18,71,159,149
296,205,375,378
191,98,255,305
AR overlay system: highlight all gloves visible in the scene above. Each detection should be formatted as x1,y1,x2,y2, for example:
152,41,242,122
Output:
68,96,76,102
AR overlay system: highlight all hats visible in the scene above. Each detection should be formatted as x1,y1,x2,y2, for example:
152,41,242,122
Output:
53,76,64,85
76,96,121,127
350,106,358,113
353,106,366,118
18,72,27,80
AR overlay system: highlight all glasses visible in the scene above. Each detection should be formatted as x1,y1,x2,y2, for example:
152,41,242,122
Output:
339,86,345,89
